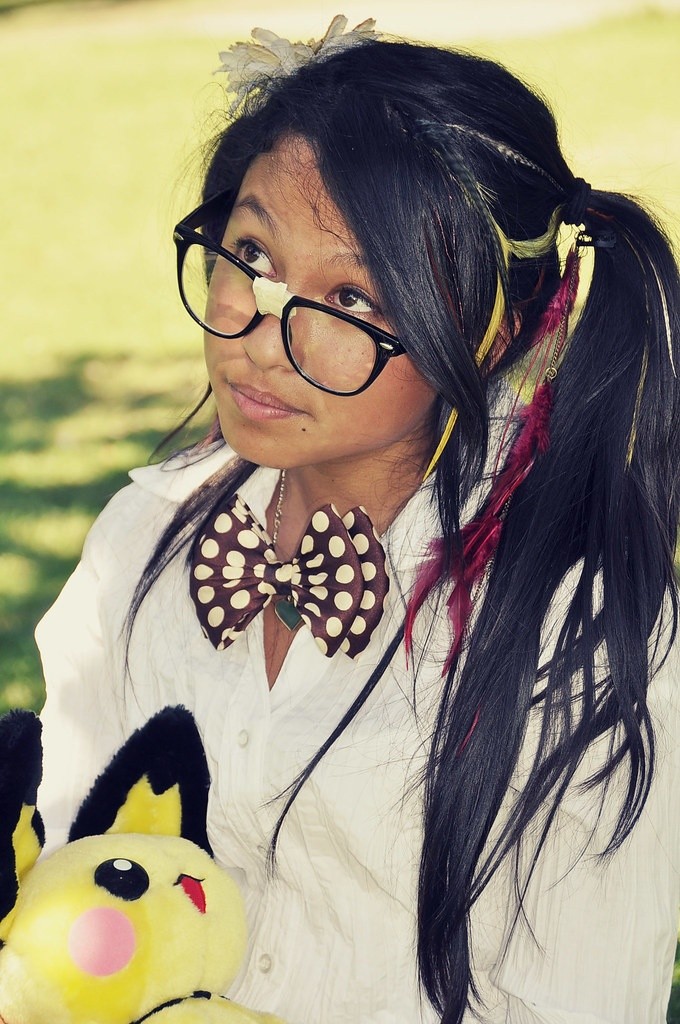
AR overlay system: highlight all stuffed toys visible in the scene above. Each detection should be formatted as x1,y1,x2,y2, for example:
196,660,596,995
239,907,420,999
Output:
0,702,279,1024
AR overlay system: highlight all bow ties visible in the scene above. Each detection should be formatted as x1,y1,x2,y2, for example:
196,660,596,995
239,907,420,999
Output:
190,493,390,660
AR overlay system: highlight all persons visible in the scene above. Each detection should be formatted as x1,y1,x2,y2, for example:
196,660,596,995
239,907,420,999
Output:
32,12,680,1024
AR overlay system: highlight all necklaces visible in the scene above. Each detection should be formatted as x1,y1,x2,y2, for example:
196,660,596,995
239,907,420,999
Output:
272,467,303,629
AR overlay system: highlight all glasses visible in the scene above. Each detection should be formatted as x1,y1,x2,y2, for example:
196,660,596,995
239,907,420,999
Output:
173,186,406,397
